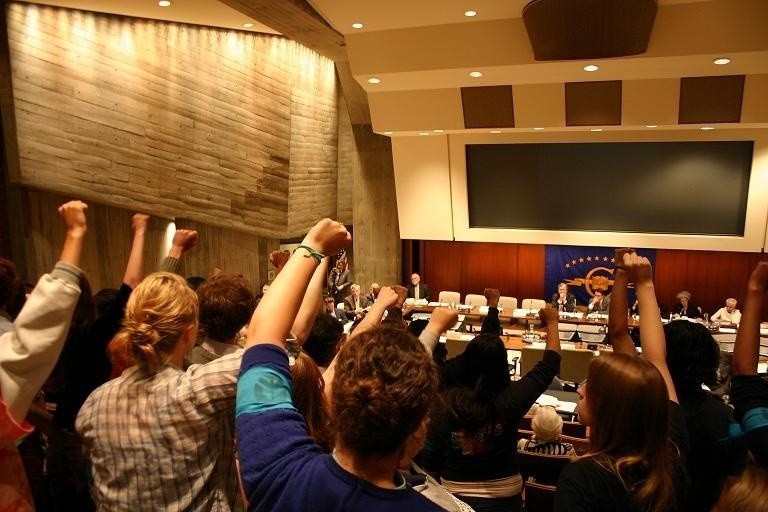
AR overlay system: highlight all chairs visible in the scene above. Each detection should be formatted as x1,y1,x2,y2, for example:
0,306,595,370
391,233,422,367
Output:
337,291,768,511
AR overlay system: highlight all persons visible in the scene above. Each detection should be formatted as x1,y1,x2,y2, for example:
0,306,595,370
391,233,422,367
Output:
1,200,768,512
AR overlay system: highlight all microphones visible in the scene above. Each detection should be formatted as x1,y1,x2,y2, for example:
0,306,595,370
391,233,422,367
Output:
527,300,537,316
